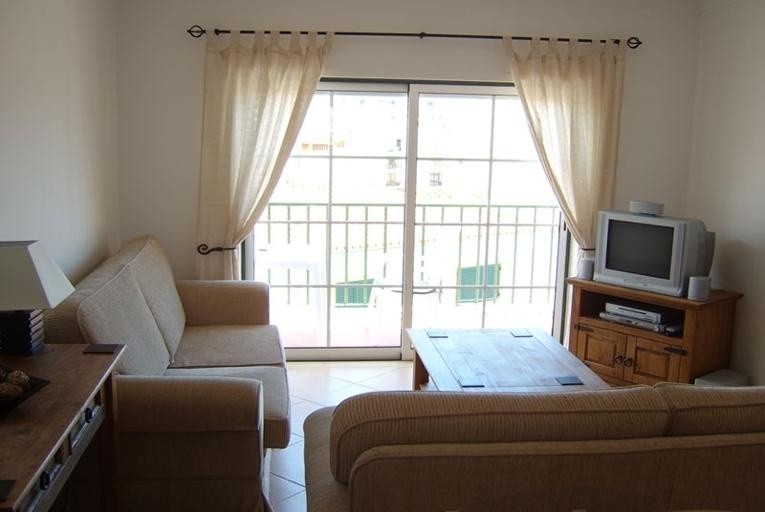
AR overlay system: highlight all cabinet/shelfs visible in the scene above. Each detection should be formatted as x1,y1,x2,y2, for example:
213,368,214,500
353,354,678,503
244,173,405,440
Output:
563,276,744,389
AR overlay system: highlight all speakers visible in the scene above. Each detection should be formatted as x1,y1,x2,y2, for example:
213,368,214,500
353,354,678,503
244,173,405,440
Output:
687,276,711,301
577,258,595,281
628,200,665,214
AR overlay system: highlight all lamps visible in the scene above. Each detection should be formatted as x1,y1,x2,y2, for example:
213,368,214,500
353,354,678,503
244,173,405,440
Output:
0,238,78,357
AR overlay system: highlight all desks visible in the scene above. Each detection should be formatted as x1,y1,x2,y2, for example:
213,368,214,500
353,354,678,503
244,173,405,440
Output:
1,341,130,511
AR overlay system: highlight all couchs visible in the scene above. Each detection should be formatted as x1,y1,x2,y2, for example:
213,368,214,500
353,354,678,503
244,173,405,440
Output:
305,383,763,509
37,236,291,512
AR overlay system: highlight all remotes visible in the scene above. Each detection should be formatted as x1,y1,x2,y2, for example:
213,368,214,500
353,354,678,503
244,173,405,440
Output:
666,325,681,332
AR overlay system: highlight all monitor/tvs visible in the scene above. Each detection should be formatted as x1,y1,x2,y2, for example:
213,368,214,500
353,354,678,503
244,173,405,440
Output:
595,211,715,298
